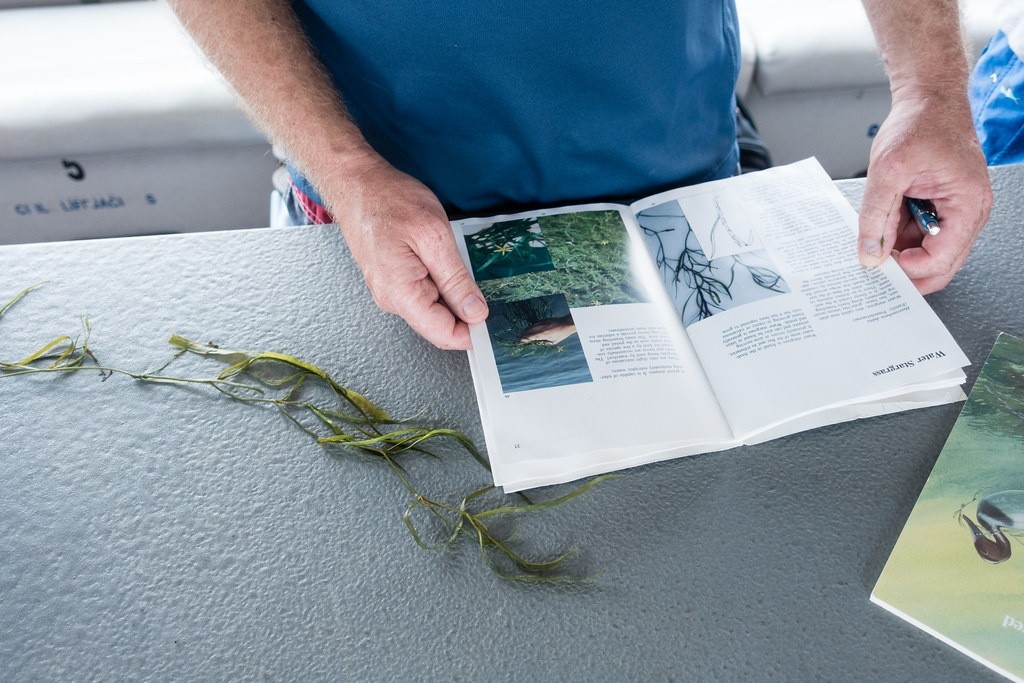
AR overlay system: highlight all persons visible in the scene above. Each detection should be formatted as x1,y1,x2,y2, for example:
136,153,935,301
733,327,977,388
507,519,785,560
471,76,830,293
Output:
520,314,576,347
166,0,993,350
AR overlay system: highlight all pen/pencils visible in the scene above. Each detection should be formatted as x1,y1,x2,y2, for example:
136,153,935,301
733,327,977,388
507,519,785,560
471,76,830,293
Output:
866,122,942,235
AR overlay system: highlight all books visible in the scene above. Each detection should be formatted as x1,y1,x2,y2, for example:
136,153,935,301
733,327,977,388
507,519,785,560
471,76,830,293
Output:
870,331,1024,683
451,157,972,495
